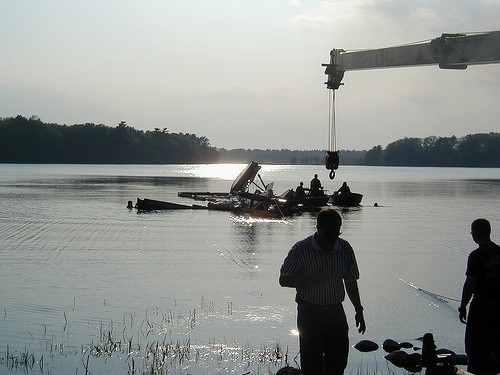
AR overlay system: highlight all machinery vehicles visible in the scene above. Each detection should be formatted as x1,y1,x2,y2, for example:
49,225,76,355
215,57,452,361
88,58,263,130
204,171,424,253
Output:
321,29,500,181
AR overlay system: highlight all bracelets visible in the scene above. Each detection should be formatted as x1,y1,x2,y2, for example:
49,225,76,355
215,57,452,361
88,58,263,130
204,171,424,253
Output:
355,306,363,313
458,307,466,312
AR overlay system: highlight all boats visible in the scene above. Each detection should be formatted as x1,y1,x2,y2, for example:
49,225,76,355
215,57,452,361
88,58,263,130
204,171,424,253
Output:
330,193,364,206
292,191,331,207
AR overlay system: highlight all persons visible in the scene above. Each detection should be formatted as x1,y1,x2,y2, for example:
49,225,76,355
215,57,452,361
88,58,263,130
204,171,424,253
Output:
459,219,500,375
310,174,323,195
334,182,352,196
296,182,307,199
279,209,367,375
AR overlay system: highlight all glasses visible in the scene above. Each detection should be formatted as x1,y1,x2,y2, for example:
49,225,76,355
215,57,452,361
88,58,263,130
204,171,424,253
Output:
324,232,341,237
